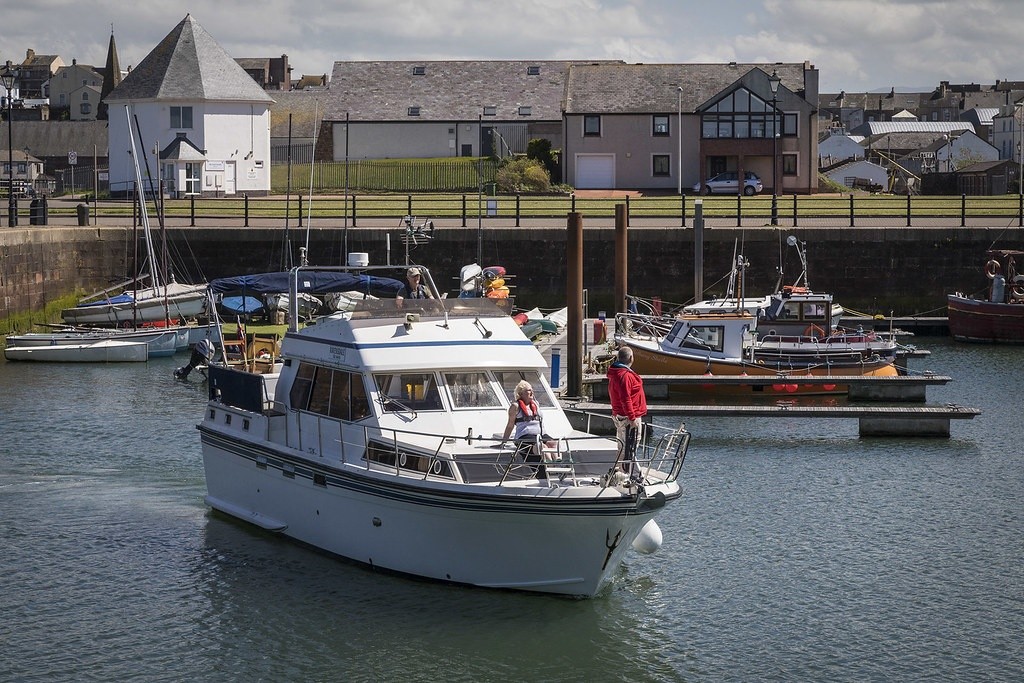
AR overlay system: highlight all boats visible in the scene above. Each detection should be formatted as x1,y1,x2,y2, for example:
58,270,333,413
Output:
618,235,925,399
944,205,1024,343
192,107,695,598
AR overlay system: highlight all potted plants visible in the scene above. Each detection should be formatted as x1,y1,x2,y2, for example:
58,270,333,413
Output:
835,112,838,117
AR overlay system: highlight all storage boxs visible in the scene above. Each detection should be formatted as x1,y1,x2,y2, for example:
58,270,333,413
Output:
270,311,285,324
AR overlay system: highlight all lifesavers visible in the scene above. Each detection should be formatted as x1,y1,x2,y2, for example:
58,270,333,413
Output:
984,260,1002,279
1009,275,1024,300
802,325,826,342
247,338,280,360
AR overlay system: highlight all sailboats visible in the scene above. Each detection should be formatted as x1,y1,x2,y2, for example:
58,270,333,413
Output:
4,106,230,363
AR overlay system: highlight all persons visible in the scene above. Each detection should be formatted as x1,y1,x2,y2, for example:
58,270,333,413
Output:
396,268,439,312
606,347,647,474
490,380,556,479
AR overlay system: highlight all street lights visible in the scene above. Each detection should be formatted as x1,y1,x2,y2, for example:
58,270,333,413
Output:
1015,103,1024,227
677,86,684,194
768,68,783,226
0,66,24,227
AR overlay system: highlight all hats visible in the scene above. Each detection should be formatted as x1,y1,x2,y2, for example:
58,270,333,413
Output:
406,267,421,278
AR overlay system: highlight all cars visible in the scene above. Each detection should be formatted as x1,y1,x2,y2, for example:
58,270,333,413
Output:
692,169,763,197
